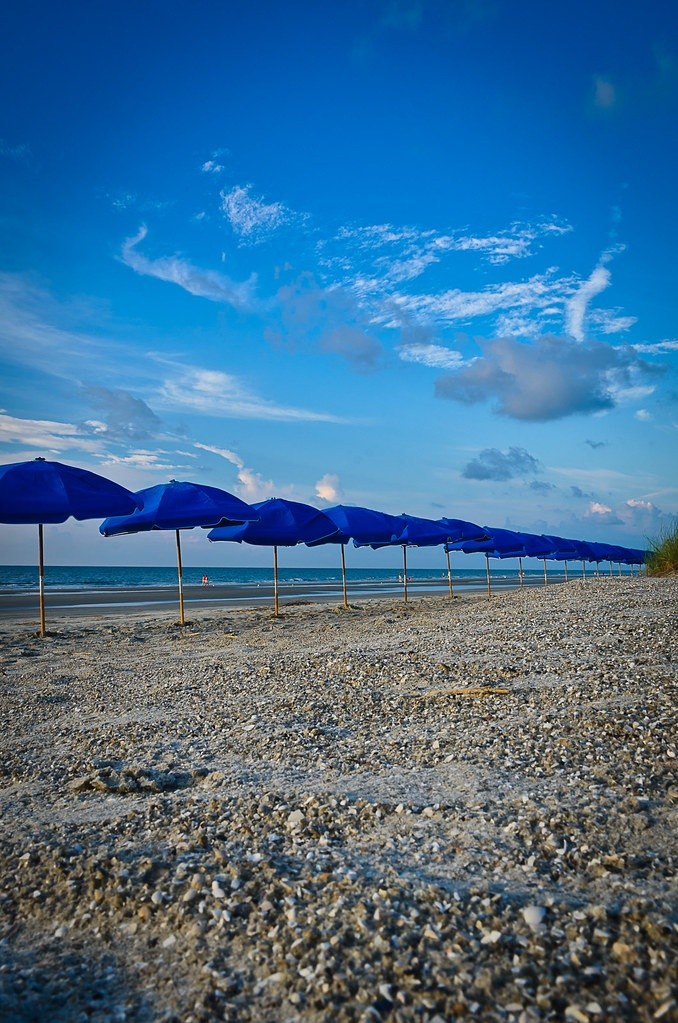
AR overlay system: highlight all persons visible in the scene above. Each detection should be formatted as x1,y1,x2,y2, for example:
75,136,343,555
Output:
201,574,209,586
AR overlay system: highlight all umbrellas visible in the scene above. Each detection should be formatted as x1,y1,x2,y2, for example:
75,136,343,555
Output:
0,457,657,638
